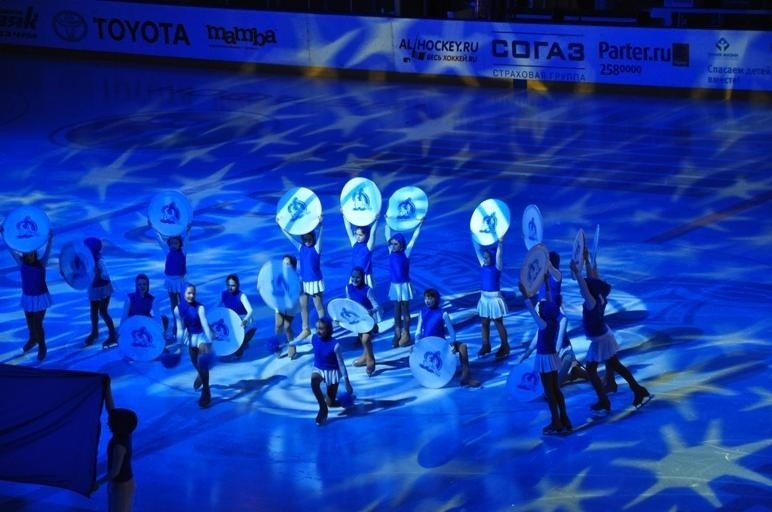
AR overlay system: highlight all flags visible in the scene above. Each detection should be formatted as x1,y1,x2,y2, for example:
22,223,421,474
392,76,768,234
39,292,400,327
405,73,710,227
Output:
1,364,109,499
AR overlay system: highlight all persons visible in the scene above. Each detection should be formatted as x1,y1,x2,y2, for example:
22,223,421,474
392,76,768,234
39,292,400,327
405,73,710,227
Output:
534,250,563,311
334,265,380,376
59,236,119,347
343,214,381,291
411,286,482,388
121,275,166,332
538,294,590,389
212,273,254,358
564,246,621,411
309,318,353,426
380,214,426,347
277,213,326,340
172,281,215,407
516,281,574,435
147,219,193,338
584,265,652,406
470,232,510,357
255,254,301,359
79,371,139,511
0,224,55,362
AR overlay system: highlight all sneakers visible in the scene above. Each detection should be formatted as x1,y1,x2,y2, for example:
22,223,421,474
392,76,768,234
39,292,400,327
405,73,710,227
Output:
274,330,311,358
543,422,575,435
633,386,650,406
193,377,210,406
495,346,509,357
591,402,612,411
315,410,327,427
391,328,410,346
477,344,491,356
352,360,367,367
462,377,480,386
103,339,116,347
38,347,47,361
366,359,375,374
602,382,618,392
23,339,36,351
86,334,98,344
